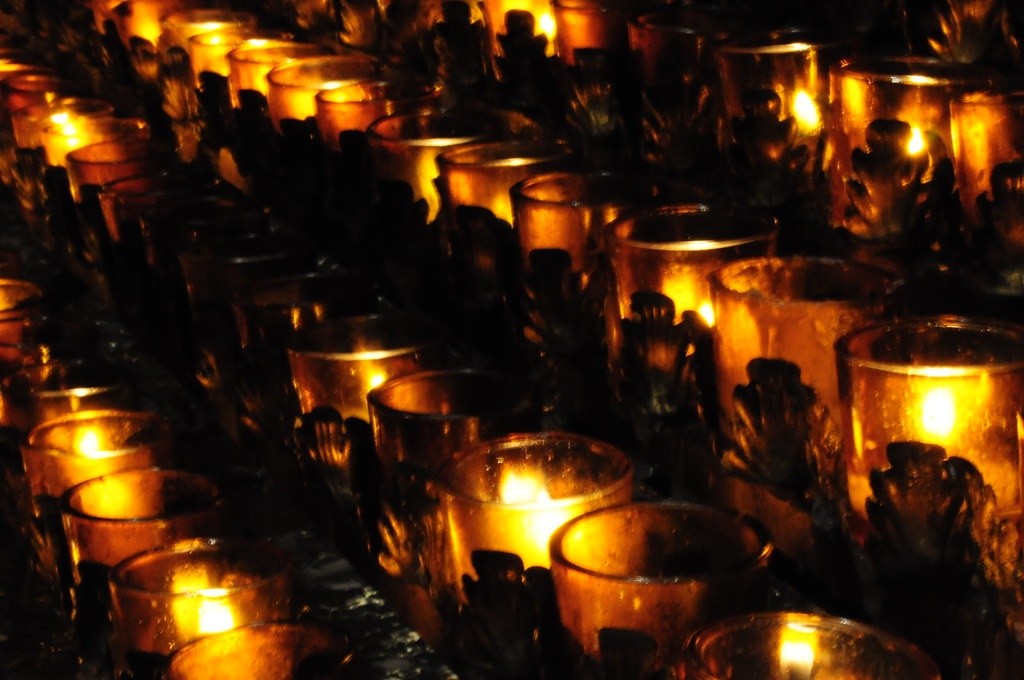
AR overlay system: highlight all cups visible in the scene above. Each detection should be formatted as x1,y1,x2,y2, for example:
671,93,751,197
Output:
0,1,1024,680
